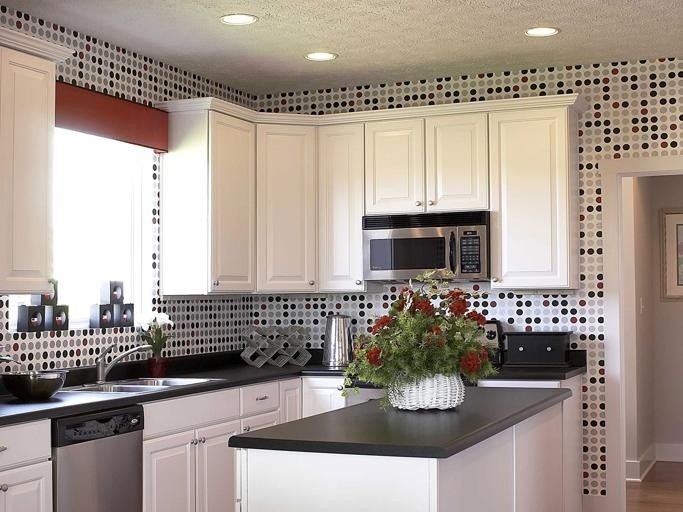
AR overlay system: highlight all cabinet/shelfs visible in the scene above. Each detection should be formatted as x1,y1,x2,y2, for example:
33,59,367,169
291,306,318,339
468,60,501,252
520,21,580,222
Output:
279,377,302,428
155,96,257,296
365,107,491,216
0,412,55,512
491,93,587,288
254,109,320,295
316,110,367,293
240,379,280,438
0,25,75,296
302,375,347,423
143,387,238,512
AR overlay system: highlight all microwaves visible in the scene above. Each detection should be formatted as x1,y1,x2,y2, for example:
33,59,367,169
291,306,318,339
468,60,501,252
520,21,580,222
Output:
361,212,489,281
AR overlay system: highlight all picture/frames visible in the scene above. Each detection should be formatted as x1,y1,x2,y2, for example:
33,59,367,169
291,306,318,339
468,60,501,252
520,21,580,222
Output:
658,206,683,303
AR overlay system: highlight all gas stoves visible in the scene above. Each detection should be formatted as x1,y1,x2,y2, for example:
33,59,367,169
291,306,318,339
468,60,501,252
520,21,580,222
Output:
347,360,502,387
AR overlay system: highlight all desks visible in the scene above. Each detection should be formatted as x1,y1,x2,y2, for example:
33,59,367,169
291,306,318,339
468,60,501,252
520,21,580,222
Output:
226,385,574,511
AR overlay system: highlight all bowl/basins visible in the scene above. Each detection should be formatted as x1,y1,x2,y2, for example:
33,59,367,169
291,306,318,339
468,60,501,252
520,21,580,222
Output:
0,369,69,402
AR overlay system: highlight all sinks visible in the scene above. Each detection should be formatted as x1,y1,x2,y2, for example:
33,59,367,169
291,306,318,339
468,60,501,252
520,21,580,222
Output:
95,376,228,386
56,383,172,395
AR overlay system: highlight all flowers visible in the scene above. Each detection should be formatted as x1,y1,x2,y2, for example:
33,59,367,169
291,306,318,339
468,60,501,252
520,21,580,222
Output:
339,258,502,417
137,309,175,358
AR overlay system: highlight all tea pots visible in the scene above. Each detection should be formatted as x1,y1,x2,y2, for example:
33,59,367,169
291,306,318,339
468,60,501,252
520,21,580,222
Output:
321,311,355,366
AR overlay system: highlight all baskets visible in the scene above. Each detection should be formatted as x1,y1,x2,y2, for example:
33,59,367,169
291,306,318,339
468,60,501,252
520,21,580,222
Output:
388,372,465,411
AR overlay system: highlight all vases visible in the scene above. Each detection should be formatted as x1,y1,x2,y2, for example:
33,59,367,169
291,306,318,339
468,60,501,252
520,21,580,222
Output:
148,355,165,377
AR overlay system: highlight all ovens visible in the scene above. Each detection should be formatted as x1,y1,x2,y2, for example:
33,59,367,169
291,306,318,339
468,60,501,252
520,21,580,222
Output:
344,388,385,408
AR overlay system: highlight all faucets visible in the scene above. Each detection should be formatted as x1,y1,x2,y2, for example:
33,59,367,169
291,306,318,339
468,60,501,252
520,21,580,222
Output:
95,342,154,384
